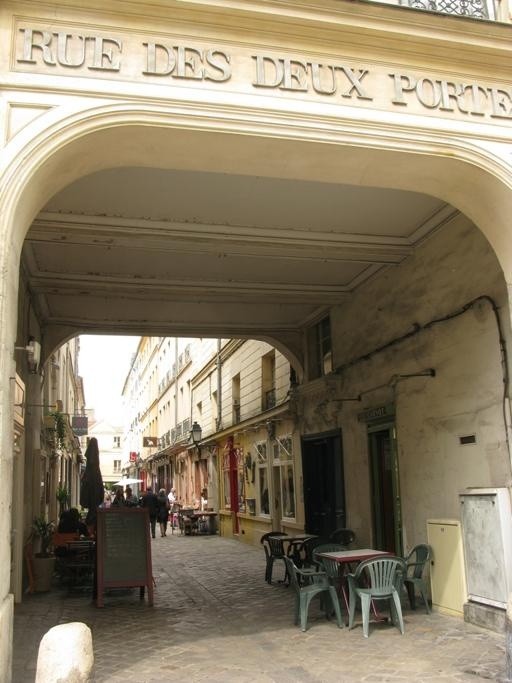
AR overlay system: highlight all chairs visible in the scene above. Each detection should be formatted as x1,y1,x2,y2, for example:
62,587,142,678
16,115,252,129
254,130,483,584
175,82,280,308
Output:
54,528,80,582
65,540,96,600
172,503,218,536
261,531,431,638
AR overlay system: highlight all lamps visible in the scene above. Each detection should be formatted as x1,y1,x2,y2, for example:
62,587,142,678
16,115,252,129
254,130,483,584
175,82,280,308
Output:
190,422,216,449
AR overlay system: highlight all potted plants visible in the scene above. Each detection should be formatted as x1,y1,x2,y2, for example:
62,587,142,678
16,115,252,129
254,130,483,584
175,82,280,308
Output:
27,515,58,592
44,411,69,453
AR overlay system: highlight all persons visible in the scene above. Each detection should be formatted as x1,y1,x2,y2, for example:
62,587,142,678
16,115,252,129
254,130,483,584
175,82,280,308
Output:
141,486,157,538
105,485,133,504
54,508,89,557
168,487,176,527
157,488,170,536
